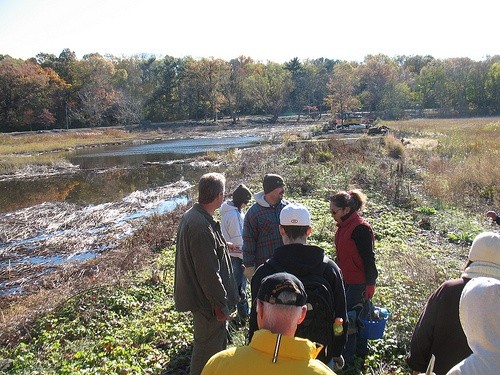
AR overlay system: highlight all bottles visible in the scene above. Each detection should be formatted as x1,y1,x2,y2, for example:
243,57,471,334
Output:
332,318,345,337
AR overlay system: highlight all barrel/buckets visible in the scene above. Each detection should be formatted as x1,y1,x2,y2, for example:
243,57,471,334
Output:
358,308,389,340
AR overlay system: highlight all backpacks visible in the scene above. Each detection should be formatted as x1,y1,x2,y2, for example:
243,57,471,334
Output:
266,258,344,364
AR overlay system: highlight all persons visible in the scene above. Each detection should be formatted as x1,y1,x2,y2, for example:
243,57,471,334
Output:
329,191,379,375
248,203,348,373
242,174,293,281
409,230,500,375
219,184,251,326
174,172,242,375
200,273,337,375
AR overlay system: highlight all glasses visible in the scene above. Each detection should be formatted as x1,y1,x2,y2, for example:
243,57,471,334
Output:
329,209,344,215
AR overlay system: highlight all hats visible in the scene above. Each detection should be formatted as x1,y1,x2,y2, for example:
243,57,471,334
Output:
279,203,311,226
233,184,251,201
468,232,500,266
263,174,285,194
253,272,313,310
